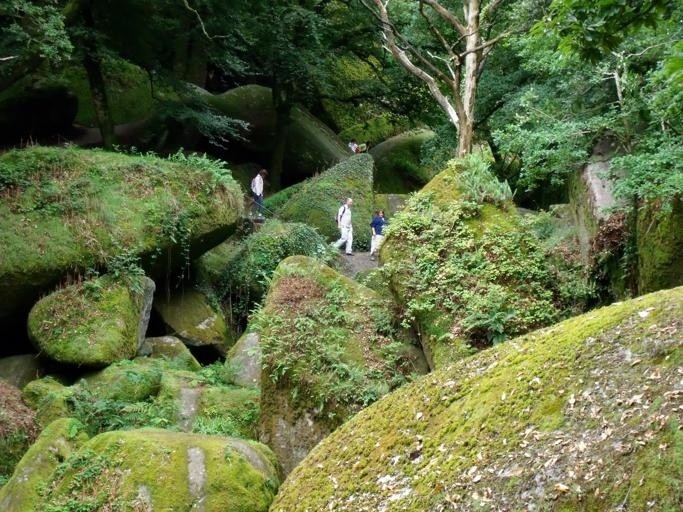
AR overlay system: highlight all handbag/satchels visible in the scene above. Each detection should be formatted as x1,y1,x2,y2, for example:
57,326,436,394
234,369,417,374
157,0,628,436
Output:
335,206,345,223
356,147,362,153
251,179,256,190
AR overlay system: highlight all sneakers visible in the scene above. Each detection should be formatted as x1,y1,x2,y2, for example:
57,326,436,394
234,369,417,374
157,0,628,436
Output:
346,253,376,260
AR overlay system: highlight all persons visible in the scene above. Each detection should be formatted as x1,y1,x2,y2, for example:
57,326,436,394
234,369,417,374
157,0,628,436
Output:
348,138,359,153
330,197,354,256
249,167,268,217
358,139,372,152
367,208,388,261
